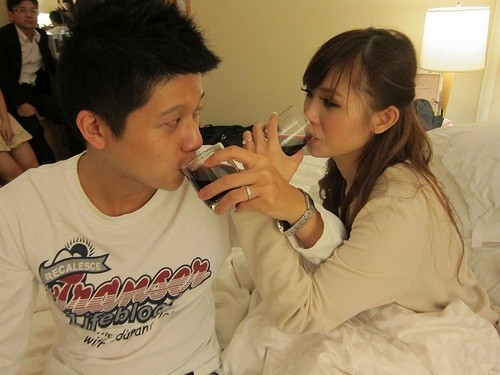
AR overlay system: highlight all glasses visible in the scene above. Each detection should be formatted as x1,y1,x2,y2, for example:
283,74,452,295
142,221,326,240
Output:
10,7,39,14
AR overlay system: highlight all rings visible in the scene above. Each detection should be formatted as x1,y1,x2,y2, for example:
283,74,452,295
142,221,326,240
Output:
245,186,252,200
242,139,253,145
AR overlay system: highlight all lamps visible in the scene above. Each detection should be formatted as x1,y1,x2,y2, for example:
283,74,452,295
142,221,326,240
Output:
418,0,490,118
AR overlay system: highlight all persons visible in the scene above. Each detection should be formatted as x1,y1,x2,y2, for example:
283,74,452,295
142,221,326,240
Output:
60,0,76,19
0,0,87,185
0,0,348,375
230,27,500,335
45,11,71,41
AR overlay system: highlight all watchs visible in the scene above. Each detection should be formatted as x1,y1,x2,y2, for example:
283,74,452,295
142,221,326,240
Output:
275,188,315,237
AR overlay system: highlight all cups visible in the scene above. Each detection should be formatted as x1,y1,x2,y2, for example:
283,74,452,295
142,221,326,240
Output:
263,105,314,156
182,142,240,210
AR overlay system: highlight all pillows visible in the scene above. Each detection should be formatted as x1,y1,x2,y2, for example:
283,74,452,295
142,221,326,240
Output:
427,115,500,249
